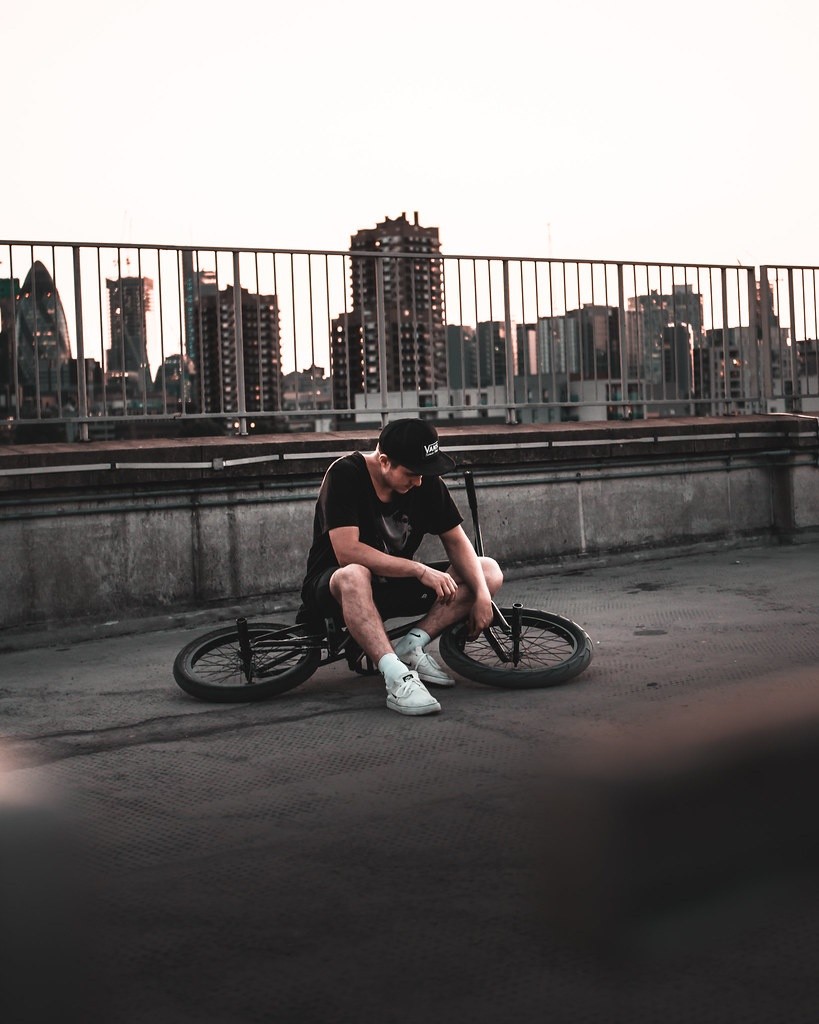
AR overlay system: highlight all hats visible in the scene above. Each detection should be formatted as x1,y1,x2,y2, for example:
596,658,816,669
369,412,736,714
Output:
379,418,457,478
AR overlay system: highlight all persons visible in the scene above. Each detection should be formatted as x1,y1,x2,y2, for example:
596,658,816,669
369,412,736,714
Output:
301,418,503,716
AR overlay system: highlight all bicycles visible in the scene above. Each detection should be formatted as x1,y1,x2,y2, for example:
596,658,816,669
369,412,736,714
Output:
171,470,596,702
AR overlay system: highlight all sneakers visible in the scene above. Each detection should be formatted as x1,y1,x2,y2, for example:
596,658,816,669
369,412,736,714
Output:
399,646,455,686
386,670,441,714
